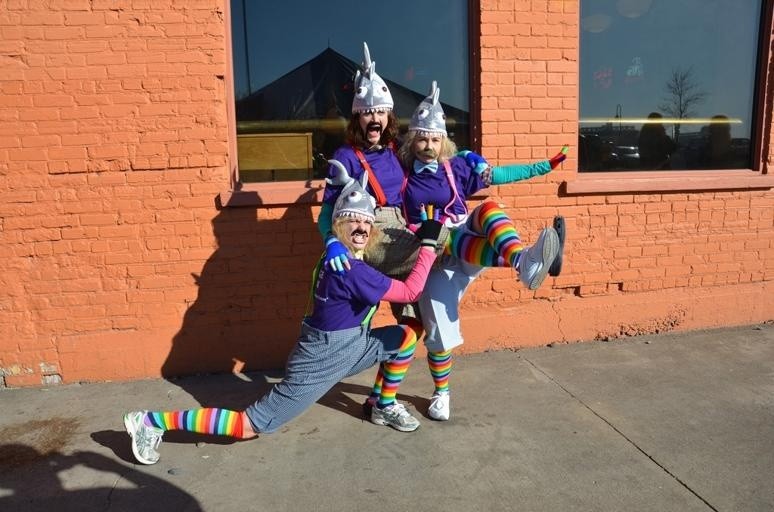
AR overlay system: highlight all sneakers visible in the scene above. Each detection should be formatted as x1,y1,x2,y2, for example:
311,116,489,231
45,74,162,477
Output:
428,391,451,421
517,228,559,290
364,393,420,432
122,410,164,465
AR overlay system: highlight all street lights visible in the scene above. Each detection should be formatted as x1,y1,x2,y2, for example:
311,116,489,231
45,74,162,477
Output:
615,103,622,136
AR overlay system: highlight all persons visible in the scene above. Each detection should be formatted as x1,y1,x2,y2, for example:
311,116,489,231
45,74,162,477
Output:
317,111,557,421
402,131,570,420
123,217,446,465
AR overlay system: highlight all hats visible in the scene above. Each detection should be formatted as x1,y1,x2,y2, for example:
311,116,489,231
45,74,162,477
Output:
352,42,394,114
325,159,377,222
409,80,447,135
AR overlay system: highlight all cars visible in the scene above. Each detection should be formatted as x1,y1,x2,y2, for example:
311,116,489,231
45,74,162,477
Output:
578,133,642,169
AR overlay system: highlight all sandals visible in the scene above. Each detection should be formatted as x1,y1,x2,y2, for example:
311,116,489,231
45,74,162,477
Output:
548,216,565,277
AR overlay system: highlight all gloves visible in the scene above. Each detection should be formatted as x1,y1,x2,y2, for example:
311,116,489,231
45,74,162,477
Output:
405,201,448,248
549,144,569,169
465,151,489,175
323,234,352,275
